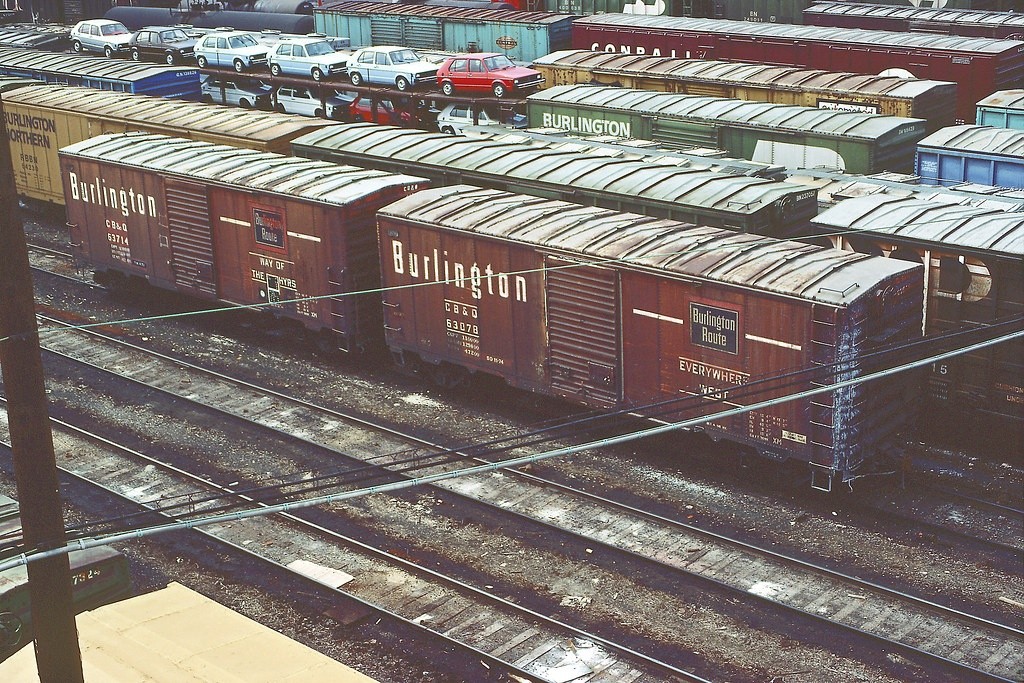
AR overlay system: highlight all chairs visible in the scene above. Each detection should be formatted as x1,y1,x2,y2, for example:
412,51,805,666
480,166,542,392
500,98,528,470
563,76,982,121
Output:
482,60,491,71
391,53,396,62
233,38,239,47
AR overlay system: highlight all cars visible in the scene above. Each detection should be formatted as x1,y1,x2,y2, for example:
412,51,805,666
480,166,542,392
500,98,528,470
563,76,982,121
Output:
270,83,355,119
436,103,527,137
128,26,197,65
345,46,440,92
266,38,351,82
200,74,273,109
436,52,546,99
70,19,133,59
192,30,272,73
348,94,437,133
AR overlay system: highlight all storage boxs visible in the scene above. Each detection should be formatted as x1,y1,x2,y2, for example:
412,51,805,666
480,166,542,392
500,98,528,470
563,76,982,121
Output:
1,0,1024,474
